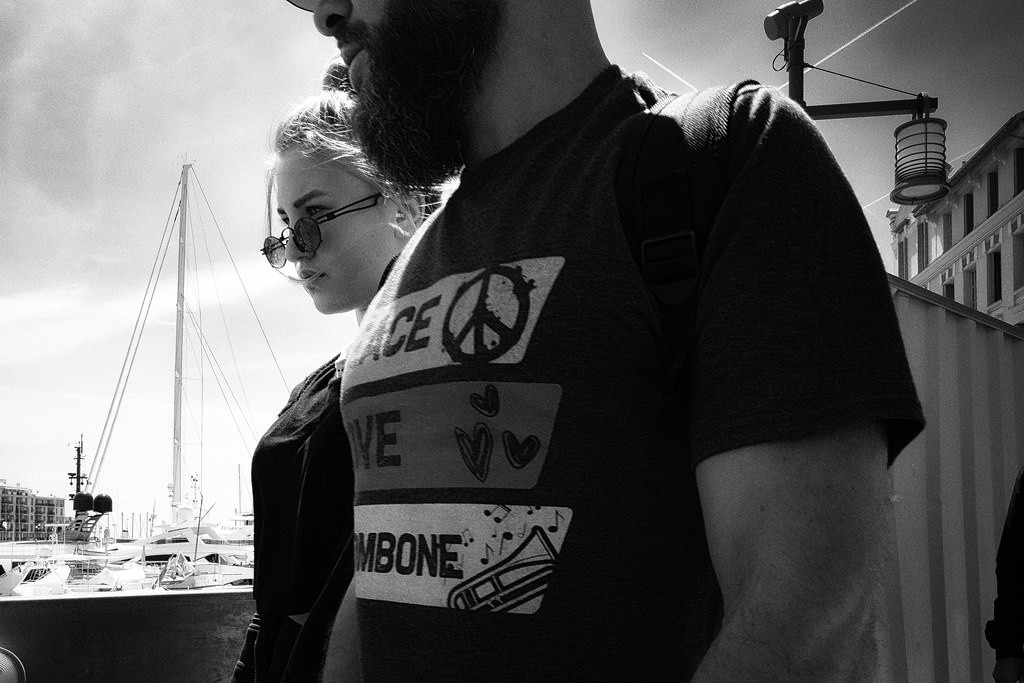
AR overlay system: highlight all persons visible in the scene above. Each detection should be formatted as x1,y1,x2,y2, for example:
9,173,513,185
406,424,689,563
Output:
984,464,1024,683
286,0,927,683
231,64,444,683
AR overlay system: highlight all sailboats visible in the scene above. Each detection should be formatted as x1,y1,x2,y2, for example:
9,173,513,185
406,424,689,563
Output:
0,150,292,598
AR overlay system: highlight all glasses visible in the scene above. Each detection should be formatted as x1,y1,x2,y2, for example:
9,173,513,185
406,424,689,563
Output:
260,190,386,269
